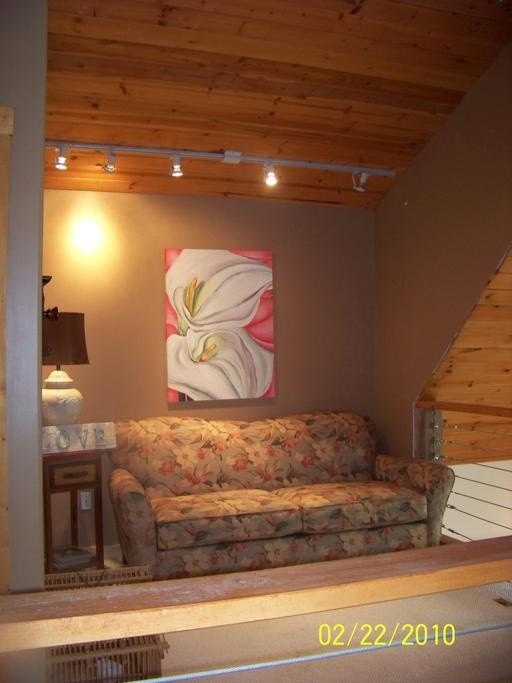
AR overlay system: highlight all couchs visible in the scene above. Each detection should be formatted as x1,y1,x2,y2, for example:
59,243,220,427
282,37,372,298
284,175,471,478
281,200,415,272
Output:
100,409,459,584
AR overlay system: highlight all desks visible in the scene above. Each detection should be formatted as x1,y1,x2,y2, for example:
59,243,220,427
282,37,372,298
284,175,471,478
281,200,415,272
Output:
41,419,120,570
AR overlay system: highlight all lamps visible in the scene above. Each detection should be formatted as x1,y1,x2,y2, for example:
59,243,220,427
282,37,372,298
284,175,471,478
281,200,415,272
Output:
39,306,97,428
40,136,398,196
